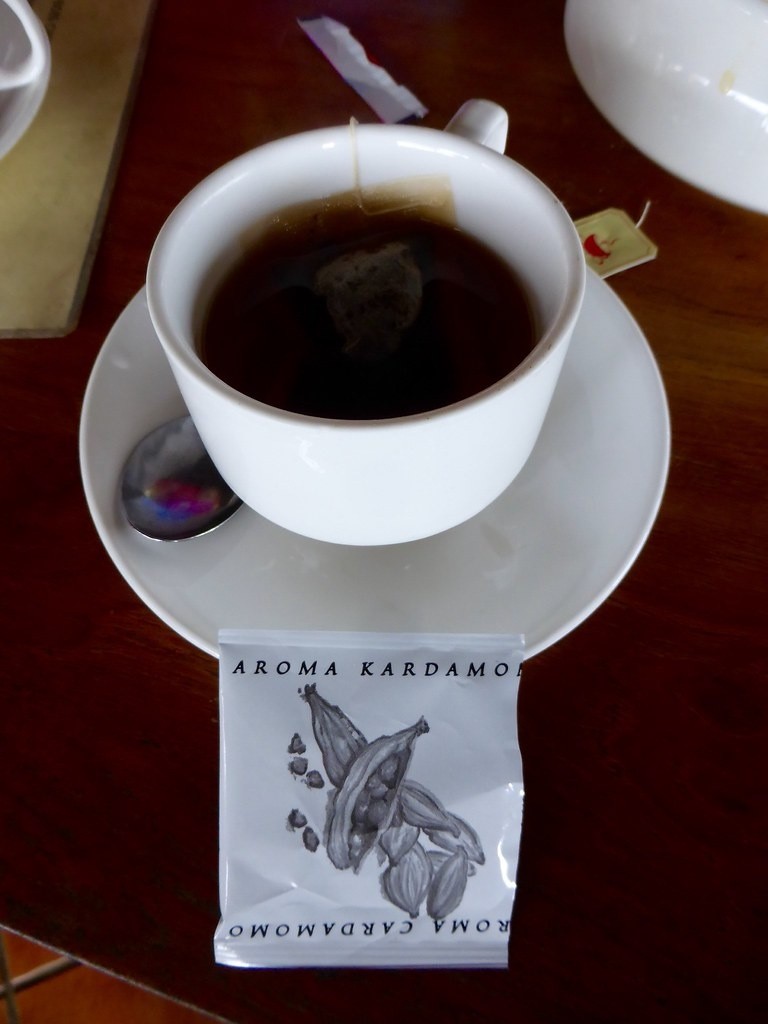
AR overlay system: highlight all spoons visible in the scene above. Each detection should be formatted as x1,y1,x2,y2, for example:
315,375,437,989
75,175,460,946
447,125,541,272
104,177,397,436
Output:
119,416,242,544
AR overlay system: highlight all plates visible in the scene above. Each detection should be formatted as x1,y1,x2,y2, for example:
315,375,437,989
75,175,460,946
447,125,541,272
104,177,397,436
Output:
0,0,50,158
561,0,768,211
76,274,675,674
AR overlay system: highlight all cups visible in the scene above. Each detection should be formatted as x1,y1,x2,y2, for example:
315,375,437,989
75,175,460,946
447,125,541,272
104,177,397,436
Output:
146,101,585,546
